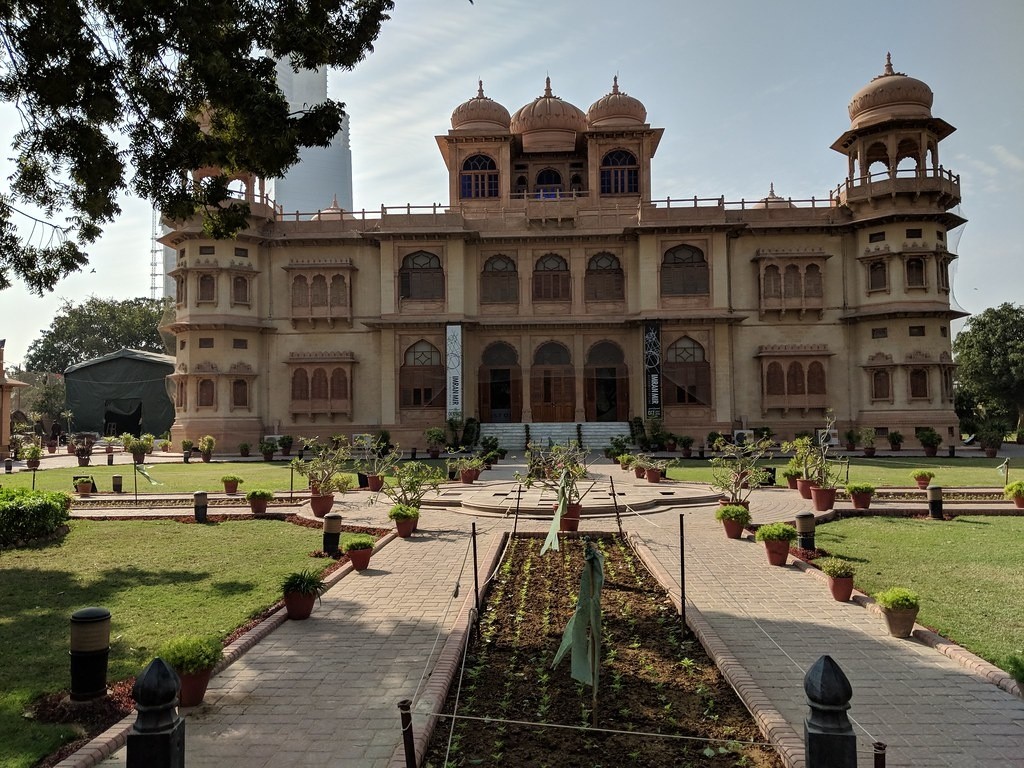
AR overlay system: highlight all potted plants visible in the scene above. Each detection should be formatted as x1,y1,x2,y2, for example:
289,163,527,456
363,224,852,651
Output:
820,556,858,603
281,568,329,621
872,585,920,639
8,427,508,518
371,460,449,530
604,416,1024,511
388,503,422,538
509,440,607,532
755,520,800,566
157,634,225,708
714,502,753,538
344,537,376,571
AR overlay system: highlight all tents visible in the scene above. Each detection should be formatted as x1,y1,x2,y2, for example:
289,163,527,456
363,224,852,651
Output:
63,346,179,440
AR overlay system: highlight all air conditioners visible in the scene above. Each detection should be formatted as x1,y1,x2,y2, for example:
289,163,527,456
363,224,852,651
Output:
352,434,371,448
734,430,755,446
818,430,838,445
263,435,284,449
